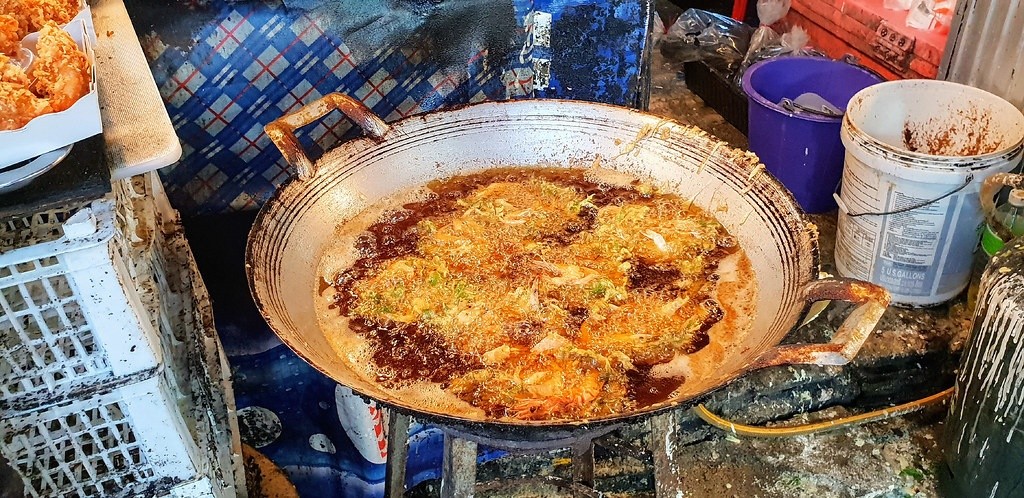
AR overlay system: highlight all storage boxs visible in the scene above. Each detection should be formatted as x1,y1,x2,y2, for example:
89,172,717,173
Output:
683,54,750,138
1,170,246,498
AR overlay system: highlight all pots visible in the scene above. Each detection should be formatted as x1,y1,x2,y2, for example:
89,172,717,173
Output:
244,93,891,427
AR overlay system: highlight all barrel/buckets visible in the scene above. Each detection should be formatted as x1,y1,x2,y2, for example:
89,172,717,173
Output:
833,79,1024,308
742,56,882,212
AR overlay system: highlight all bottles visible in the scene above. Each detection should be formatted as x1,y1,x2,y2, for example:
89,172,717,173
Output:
967,189,1024,312
